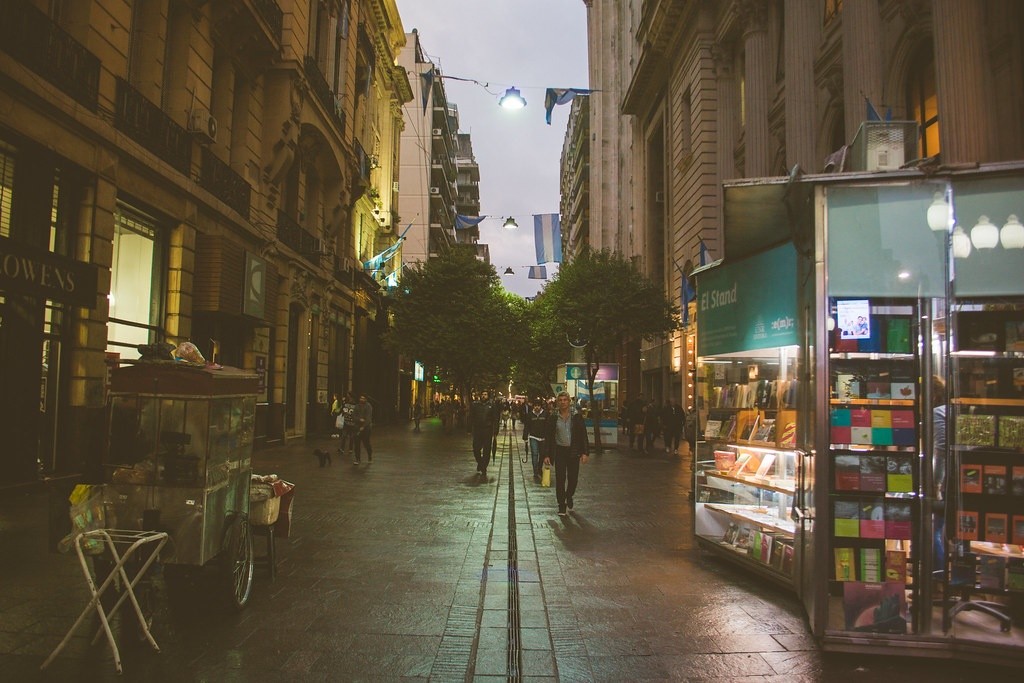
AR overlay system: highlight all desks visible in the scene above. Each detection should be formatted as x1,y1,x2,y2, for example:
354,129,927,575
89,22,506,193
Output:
970,541,1024,598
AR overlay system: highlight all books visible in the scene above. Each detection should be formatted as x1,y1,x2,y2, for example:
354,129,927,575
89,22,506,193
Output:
747,527,796,577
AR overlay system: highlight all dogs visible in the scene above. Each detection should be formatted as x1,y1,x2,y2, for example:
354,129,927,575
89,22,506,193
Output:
312,449,331,468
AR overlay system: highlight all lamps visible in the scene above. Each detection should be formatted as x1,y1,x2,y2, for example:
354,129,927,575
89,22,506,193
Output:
379,226,390,229
374,218,384,222
371,210,379,214
360,262,385,271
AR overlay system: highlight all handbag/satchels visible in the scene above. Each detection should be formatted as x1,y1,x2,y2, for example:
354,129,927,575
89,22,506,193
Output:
541,463,551,486
633,423,644,434
335,413,344,429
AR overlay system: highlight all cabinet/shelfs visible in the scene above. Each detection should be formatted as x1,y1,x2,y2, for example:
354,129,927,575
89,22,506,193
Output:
826,303,920,635
694,306,810,598
942,300,1024,634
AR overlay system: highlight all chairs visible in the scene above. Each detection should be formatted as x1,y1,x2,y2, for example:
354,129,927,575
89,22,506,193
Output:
933,551,977,605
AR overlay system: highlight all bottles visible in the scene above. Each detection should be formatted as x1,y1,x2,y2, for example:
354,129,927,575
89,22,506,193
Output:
57,532,70,554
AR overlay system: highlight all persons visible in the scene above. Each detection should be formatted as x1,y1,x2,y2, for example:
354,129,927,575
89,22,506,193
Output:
328,393,375,466
544,390,591,518
413,397,466,432
522,400,548,482
468,388,504,478
491,393,528,430
622,389,687,457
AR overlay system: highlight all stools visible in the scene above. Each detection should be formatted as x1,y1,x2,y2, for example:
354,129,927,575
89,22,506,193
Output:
249,526,276,581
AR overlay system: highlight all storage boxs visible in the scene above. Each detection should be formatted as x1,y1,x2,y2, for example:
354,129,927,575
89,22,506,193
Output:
835,548,855,582
247,485,280,525
834,363,915,400
1011,515,1024,545
831,406,915,447
955,414,997,445
984,513,1008,544
860,549,881,584
1005,320,1024,353
955,510,980,541
884,551,906,582
833,497,912,539
998,416,1024,446
833,315,911,353
960,464,1024,497
842,582,905,625
834,456,913,492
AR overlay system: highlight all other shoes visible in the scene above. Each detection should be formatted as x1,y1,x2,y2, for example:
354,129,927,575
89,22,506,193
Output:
644,449,648,454
477,463,481,470
674,450,678,455
339,448,344,453
665,448,669,452
568,496,573,508
558,505,566,516
349,450,352,453
353,461,360,464
482,470,486,476
369,460,372,464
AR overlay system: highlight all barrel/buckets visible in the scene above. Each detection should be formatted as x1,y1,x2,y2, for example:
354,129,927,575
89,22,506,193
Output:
249,483,280,525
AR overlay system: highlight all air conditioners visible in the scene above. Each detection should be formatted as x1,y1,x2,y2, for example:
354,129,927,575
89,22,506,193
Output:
430,187,439,194
338,257,349,273
433,129,441,137
393,182,399,192
433,159,442,165
866,125,906,173
188,107,217,144
